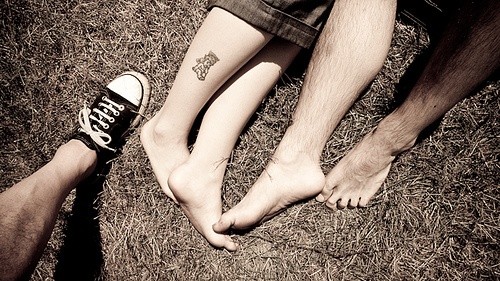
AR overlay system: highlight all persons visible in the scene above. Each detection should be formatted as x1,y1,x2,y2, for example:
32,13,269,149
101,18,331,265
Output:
213,0,500,232
1,72,151,281
141,0,336,252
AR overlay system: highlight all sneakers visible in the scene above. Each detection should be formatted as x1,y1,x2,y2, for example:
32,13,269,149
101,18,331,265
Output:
70,68,151,187
